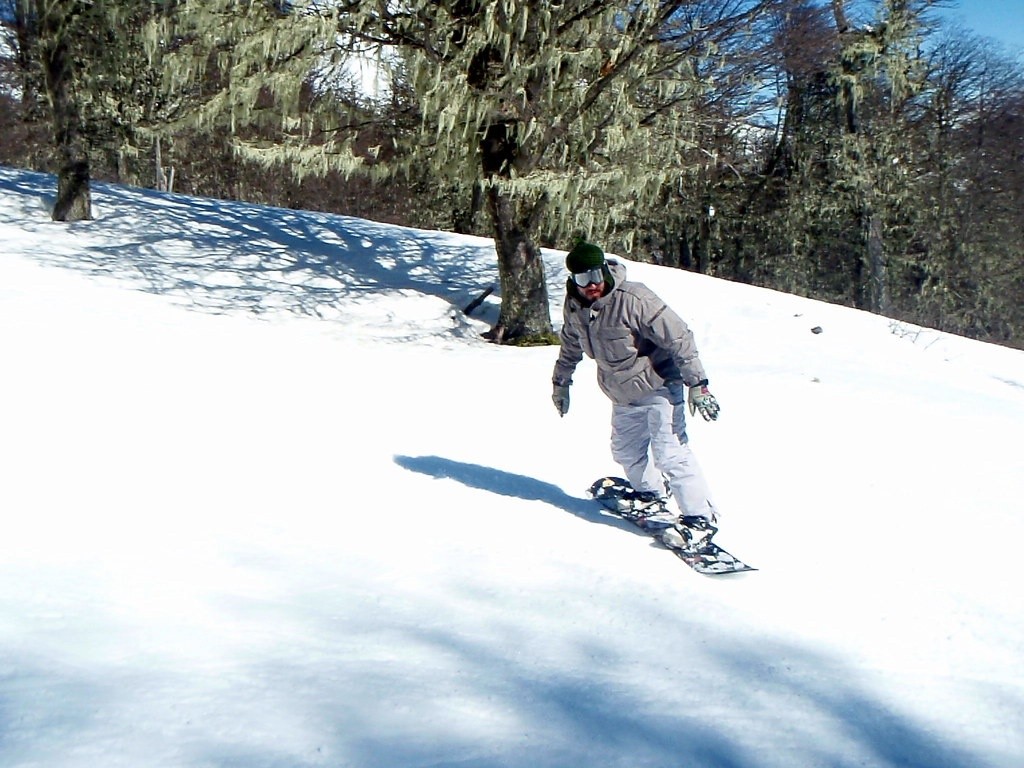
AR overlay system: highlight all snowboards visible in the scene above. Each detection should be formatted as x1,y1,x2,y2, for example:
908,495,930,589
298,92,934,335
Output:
590,475,762,577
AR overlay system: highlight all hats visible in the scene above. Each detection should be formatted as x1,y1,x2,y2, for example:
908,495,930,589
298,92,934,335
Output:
566,241,603,273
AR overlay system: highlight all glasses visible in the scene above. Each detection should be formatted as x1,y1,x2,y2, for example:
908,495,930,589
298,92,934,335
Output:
573,267,605,287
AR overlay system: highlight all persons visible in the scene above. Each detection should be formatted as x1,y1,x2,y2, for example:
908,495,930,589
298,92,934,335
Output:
552,241,722,547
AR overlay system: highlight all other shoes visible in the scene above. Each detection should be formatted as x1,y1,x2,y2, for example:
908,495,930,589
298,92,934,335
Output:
617,495,660,512
663,515,716,548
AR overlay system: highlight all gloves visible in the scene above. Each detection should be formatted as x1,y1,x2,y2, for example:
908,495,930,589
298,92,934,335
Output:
688,384,720,421
552,385,570,417
